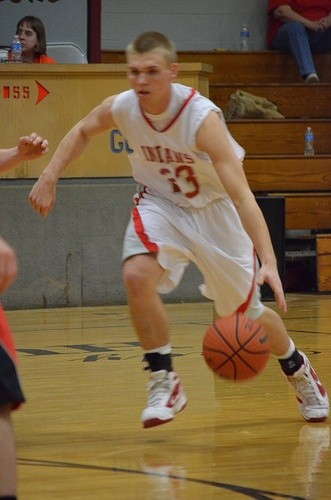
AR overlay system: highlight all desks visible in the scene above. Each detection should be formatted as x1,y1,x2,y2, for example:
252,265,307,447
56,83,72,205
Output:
0,62,213,179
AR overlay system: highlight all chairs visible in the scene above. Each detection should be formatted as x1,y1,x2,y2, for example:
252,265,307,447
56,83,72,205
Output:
46,42,88,64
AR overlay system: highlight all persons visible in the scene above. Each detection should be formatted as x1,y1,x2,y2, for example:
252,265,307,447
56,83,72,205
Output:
0,15,57,500
29,32,331,430
266,0,330,84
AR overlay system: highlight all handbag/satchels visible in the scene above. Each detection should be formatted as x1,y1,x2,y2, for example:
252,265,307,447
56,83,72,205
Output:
225,90,284,119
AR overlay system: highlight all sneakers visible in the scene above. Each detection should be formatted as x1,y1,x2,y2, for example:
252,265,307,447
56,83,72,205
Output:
280,349,328,421
141,369,187,429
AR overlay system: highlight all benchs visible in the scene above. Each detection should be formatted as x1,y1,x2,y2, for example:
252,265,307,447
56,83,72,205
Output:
99,49,331,294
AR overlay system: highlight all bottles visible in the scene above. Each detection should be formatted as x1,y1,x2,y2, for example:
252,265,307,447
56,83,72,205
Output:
240,24,249,50
11,35,21,63
304,127,314,155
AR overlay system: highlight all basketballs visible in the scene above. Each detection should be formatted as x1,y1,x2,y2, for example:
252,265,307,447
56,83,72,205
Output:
201,312,271,382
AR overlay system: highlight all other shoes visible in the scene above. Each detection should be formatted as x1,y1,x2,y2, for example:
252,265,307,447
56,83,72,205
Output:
305,73,320,83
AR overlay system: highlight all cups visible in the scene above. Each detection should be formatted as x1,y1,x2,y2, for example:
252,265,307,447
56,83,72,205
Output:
0,49,9,63
